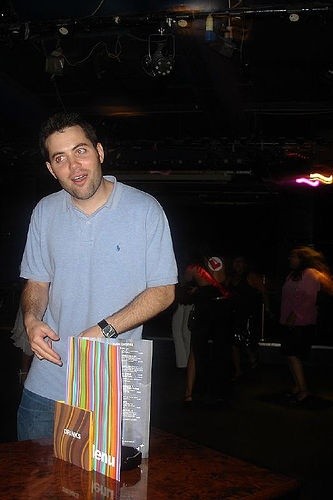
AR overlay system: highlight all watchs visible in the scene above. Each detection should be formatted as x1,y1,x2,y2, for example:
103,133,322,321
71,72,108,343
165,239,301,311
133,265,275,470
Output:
97,318,118,338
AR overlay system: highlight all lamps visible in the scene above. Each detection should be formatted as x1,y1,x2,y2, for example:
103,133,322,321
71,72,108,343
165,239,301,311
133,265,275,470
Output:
54,40,84,68
214,25,244,55
147,34,175,77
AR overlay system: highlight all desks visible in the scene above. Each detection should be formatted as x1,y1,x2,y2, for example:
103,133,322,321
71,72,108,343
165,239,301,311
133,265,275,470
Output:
0,426,301,500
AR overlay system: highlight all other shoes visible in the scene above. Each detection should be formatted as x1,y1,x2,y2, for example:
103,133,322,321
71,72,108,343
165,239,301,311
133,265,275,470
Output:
288,393,314,404
183,395,193,406
278,391,301,401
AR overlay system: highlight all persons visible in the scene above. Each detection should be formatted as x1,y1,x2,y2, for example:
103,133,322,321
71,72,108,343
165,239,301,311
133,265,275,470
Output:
170,243,320,409
15,111,178,443
11,305,34,387
